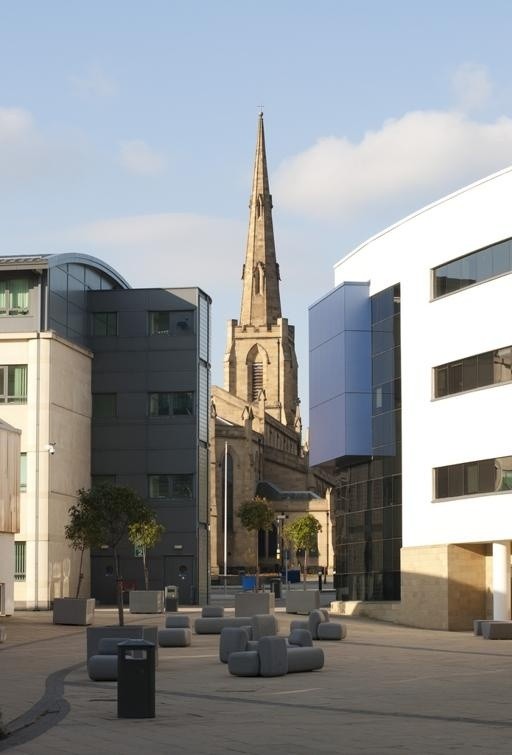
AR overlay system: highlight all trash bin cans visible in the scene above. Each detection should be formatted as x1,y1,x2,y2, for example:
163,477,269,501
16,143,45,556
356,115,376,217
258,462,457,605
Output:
289,570,300,583
270,578,281,598
165,586,177,612
242,575,256,593
117,639,156,719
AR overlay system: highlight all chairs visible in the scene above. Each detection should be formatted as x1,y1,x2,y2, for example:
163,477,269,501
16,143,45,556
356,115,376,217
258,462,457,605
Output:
86,604,348,683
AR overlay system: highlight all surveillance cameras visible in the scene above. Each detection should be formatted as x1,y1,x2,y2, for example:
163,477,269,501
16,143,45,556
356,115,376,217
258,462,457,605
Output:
50,449,56,455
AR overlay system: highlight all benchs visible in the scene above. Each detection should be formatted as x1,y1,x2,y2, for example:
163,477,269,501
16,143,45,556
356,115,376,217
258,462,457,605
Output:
472,618,512,641
